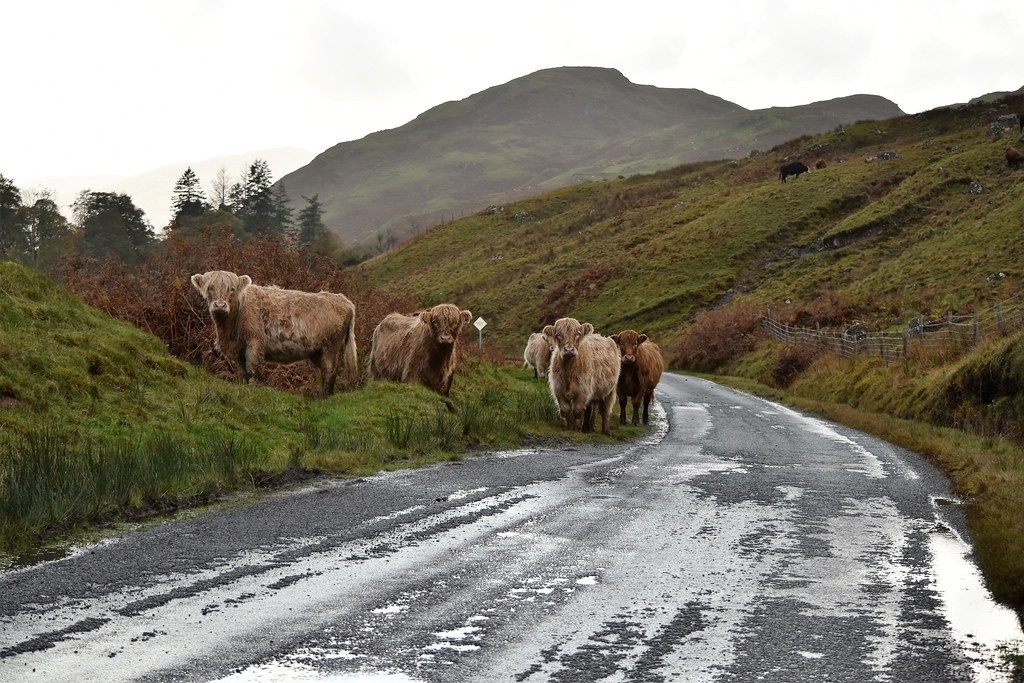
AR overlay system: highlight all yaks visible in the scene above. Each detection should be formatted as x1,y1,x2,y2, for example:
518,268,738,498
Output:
370,303,474,397
524,315,665,436
191,271,358,400
